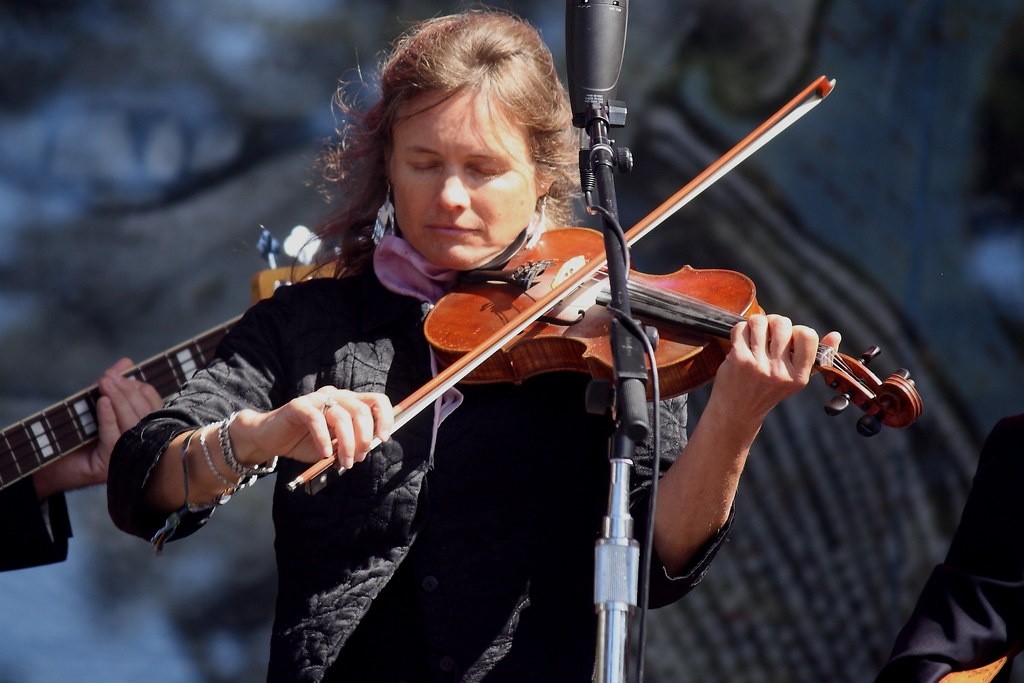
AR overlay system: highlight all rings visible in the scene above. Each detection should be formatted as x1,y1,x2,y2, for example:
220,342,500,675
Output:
322,399,337,415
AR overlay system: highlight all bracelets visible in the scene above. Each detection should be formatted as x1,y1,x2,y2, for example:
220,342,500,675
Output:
148,409,278,556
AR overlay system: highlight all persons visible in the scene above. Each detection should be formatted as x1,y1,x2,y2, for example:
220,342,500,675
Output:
106,13,843,683
882,419,1023,683
0,358,162,576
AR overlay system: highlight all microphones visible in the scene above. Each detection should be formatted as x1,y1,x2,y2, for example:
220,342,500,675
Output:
566,0,630,125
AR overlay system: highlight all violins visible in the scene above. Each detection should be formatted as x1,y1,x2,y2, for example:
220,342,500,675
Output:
423,227,925,438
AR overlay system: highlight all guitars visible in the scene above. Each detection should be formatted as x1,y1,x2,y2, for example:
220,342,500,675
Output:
0,225,344,491
